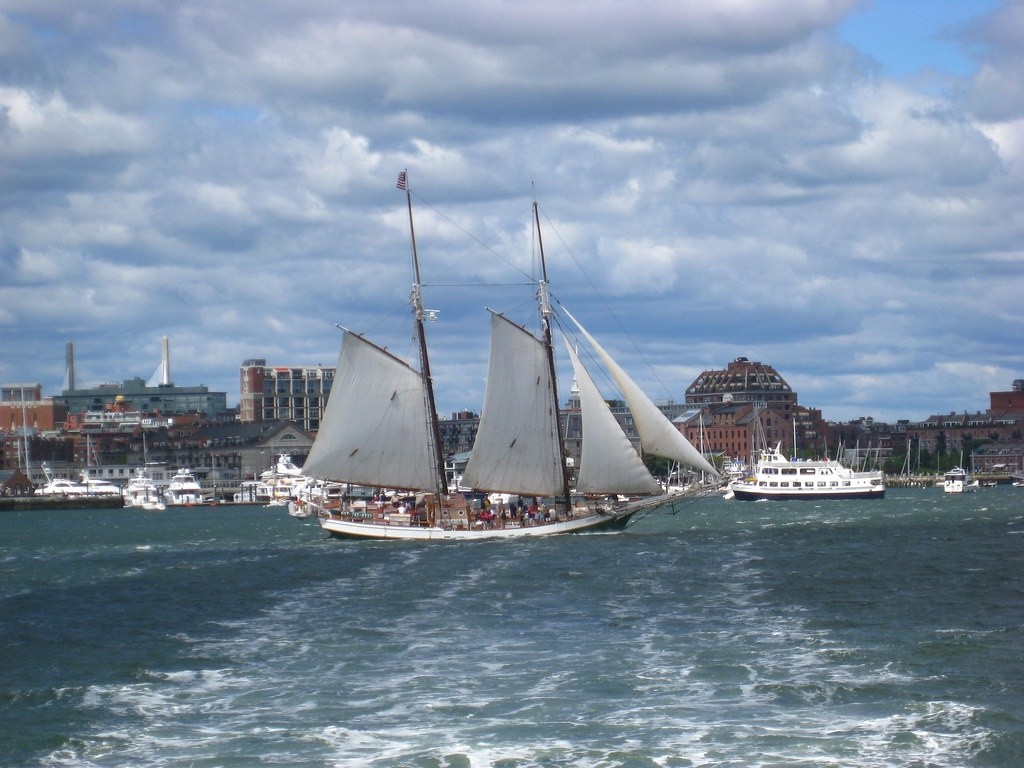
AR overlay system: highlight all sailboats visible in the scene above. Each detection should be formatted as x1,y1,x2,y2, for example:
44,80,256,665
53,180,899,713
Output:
300,168,728,541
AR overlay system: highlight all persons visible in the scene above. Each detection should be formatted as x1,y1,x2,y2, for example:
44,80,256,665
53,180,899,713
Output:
510,503,517,518
371,491,386,503
390,492,410,515
518,495,551,526
499,509,507,530
533,496,539,512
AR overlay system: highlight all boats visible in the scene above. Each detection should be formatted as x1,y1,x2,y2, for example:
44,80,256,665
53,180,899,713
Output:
233,454,325,503
34,478,89,497
81,480,126,497
1013,480,1024,487
730,441,886,501
163,468,202,507
122,462,166,510
944,469,979,493
